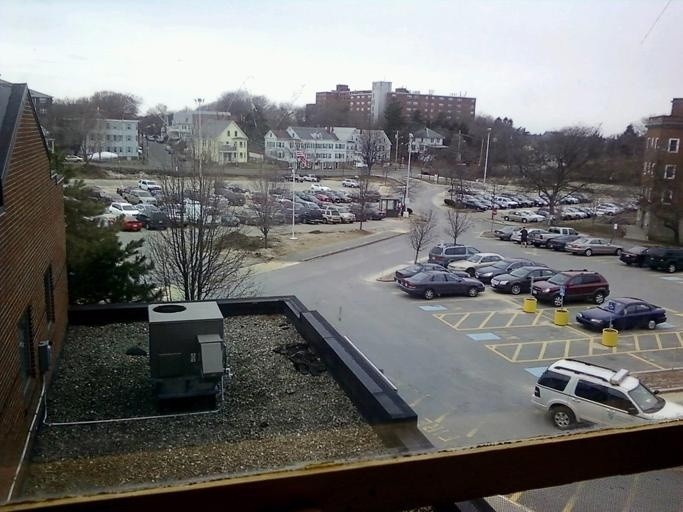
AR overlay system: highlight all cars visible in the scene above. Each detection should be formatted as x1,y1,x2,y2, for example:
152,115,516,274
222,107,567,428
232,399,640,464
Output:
576,296,663,332
444,186,641,223
64,154,83,163
620,245,650,269
89,186,402,232
550,235,582,250
494,225,522,241
447,252,504,278
393,264,470,286
137,134,187,162
508,228,544,246
564,237,623,258
474,258,539,284
398,269,484,300
490,265,556,296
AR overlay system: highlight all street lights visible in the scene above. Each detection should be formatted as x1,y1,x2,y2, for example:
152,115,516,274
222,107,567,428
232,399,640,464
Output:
483,126,492,184
193,96,205,176
404,132,413,218
286,157,298,241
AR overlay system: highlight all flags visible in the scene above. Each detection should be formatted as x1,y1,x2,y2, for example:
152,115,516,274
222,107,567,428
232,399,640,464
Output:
297,148,308,167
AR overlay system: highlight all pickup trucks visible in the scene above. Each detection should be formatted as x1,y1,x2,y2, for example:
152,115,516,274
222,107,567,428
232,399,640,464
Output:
532,226,576,248
342,179,360,188
303,174,317,182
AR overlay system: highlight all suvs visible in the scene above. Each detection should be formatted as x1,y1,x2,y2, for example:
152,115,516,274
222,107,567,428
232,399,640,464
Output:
646,248,682,275
529,357,682,430
531,270,609,307
141,180,162,191
428,242,482,267
138,179,143,187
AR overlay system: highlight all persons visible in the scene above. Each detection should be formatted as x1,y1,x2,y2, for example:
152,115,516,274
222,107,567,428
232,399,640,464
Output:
519,225,529,248
491,205,498,223
